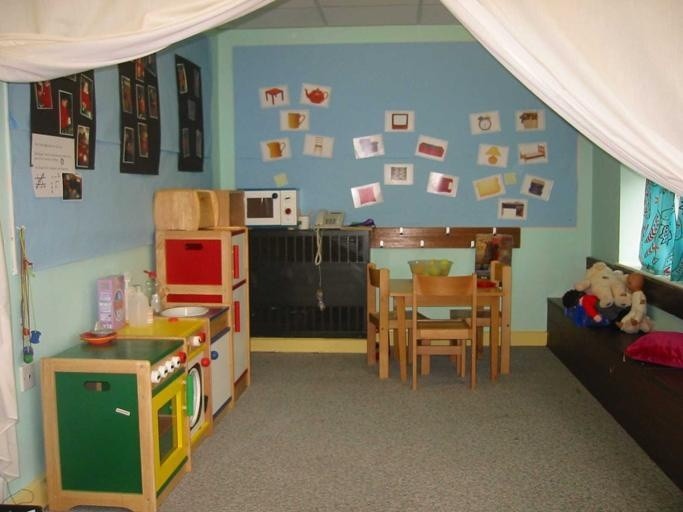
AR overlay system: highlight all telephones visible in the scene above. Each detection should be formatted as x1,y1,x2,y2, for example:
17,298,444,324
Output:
312,210,345,229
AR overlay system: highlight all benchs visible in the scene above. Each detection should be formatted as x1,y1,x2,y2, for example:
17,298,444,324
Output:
547,257,683,491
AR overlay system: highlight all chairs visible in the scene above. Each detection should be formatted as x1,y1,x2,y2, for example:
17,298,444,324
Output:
449,261,511,374
412,272,477,391
367,263,430,379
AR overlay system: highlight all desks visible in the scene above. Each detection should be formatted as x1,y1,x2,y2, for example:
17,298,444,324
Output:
40,337,192,512
153,305,232,427
114,318,214,456
386,276,506,379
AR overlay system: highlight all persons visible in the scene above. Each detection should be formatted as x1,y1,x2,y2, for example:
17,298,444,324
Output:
121,55,158,161
38,80,52,108
60,96,71,130
78,132,89,166
81,80,91,114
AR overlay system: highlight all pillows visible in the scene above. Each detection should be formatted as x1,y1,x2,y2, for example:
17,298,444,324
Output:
623,332,683,368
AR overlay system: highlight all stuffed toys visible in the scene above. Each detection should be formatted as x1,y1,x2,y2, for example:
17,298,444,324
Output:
560,262,650,333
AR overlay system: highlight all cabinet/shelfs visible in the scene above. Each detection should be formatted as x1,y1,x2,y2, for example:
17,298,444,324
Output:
156,227,252,407
